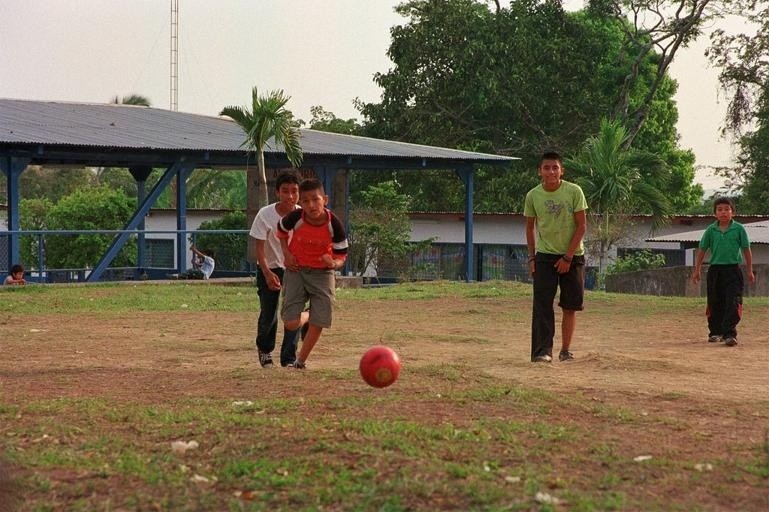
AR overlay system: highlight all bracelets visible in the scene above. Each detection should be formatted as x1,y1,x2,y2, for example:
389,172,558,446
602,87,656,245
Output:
562,255,572,262
528,255,536,262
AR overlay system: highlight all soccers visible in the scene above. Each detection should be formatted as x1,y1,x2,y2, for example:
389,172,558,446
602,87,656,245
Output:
361,347,400,388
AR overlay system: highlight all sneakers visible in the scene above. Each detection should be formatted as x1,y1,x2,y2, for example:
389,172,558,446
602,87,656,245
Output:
294,359,306,369
535,352,552,362
709,336,721,341
559,352,573,362
726,337,737,344
259,348,272,369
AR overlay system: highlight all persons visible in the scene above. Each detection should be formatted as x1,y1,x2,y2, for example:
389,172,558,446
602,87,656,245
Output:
248,169,304,368
275,177,349,369
523,150,589,362
3,265,26,285
166,245,215,280
692,196,755,345
